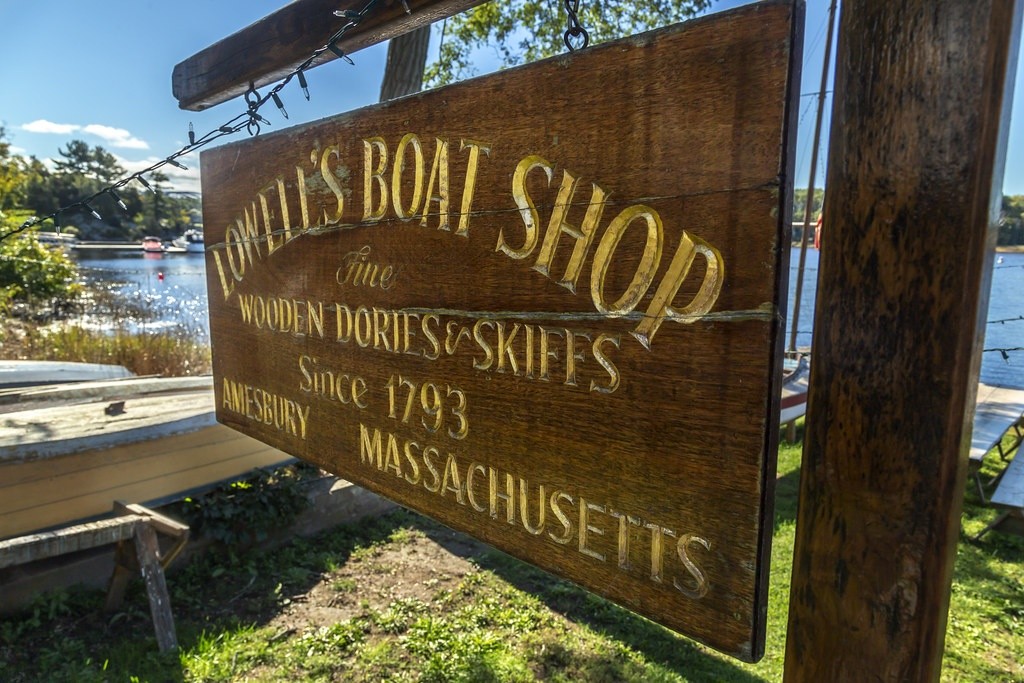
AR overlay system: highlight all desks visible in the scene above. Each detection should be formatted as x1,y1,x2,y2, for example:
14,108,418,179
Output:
968,400,1024,510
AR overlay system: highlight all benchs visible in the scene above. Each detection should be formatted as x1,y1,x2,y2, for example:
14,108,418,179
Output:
990,439,1024,535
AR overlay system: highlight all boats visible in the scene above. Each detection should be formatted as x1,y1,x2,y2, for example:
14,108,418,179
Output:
142,237,163,251
172,228,204,253
